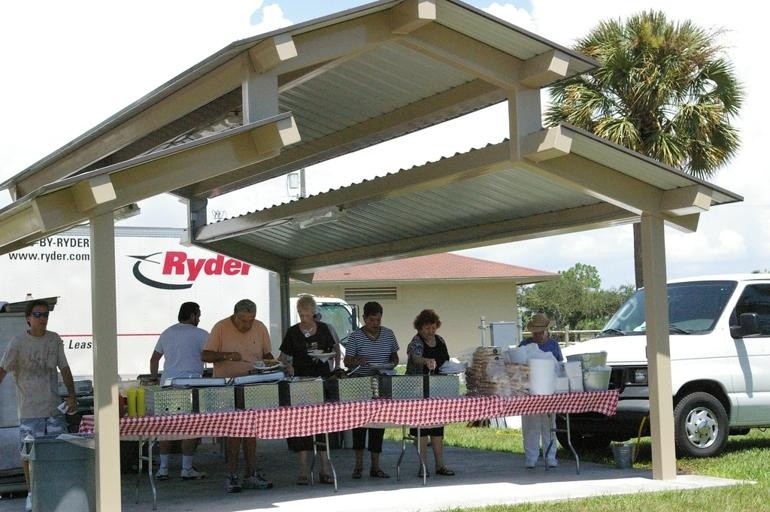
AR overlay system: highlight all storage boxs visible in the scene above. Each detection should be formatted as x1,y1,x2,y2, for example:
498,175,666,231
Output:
278,376,324,408
193,385,235,414
379,374,423,401
566,350,607,372
424,372,467,398
324,375,379,403
235,382,280,410
145,385,193,417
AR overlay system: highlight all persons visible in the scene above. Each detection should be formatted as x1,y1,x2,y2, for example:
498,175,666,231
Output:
344,302,400,479
406,309,455,477
519,315,563,468
151,302,208,481
0,301,76,511
275,305,344,448
280,297,340,485
201,300,274,492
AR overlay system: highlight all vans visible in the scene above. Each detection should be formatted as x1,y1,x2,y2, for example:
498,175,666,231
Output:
556,273,770,457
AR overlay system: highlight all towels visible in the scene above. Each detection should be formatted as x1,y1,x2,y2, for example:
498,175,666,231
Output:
439,361,466,372
314,354,332,362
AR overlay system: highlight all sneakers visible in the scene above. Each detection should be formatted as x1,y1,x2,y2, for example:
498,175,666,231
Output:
25,492,33,512
155,467,169,480
319,472,334,484
224,471,242,493
181,467,208,480
296,472,309,485
525,460,536,468
541,459,557,467
241,468,273,489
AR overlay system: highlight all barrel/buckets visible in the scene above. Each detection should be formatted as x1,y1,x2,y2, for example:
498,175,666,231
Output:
610,440,633,472
567,352,607,395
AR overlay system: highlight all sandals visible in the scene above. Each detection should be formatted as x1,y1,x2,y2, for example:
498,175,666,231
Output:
352,468,364,479
370,469,392,478
435,465,454,475
418,465,429,477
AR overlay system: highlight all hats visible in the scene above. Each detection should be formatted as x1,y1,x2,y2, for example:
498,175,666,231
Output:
527,314,550,333
314,307,322,315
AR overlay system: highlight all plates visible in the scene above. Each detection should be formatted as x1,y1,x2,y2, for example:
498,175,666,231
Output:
284,376,317,382
438,370,466,374
252,359,282,370
308,352,337,358
368,363,395,369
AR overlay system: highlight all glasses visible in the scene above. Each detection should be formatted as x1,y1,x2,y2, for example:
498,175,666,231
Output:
32,312,49,318
237,315,255,323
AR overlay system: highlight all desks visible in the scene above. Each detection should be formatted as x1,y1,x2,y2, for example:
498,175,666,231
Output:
78,388,620,512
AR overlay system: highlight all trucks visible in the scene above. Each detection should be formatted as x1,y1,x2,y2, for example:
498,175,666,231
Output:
0,222,362,463
